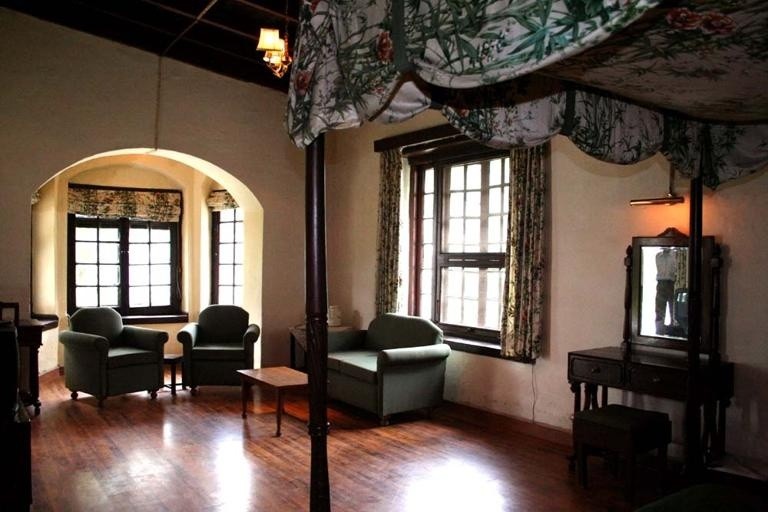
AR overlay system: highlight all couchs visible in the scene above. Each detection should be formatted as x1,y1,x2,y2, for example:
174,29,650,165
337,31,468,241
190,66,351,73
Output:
327,314,450,427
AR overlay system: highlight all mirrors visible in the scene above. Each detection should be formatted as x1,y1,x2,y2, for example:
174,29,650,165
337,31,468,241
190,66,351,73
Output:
626,226,723,365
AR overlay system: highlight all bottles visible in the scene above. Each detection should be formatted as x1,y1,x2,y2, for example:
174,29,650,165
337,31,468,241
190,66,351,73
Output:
327,306,342,327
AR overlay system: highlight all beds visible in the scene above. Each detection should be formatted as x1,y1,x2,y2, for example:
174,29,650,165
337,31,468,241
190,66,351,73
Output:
279,0,766,511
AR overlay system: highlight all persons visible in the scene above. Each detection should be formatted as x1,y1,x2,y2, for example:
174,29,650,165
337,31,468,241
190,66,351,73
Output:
653,246,678,335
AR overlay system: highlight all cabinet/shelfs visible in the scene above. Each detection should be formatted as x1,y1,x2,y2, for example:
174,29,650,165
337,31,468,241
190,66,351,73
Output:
567,343,734,502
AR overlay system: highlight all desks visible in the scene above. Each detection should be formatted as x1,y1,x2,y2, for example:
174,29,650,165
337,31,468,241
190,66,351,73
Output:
287,325,352,372
0,313,58,512
234,365,309,438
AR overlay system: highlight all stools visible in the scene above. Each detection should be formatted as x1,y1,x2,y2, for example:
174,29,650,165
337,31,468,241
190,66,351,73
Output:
570,404,671,504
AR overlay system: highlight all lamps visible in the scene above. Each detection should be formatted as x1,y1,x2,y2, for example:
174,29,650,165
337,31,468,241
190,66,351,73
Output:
254,0,295,78
628,165,684,208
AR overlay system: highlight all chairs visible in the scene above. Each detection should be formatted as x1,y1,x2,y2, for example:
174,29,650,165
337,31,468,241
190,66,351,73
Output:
55,305,261,424
